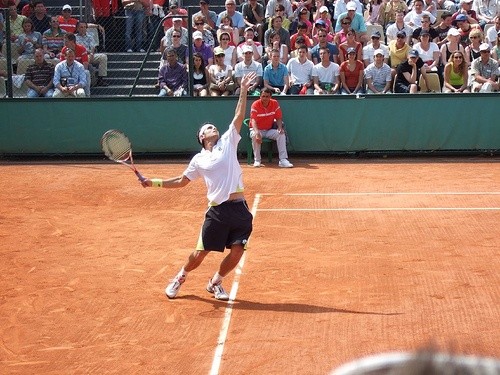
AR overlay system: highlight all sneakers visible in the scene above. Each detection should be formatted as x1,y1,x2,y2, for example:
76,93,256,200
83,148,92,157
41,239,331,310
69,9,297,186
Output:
253,160,261,166
206,278,229,299
97,79,108,86
139,49,145,52
127,49,132,53
165,275,186,299
279,159,293,168
155,83,160,88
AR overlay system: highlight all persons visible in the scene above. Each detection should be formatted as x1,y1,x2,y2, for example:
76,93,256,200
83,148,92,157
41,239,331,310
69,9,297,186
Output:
470,43,500,93
264,15,290,52
289,22,313,53
433,12,458,40
412,30,440,72
265,0,294,23
191,0,217,32
333,0,363,28
411,14,440,46
362,30,389,68
364,48,392,94
311,28,338,65
185,30,214,69
145,0,165,54
395,49,430,94
192,15,215,49
121,0,150,53
208,46,233,97
370,0,389,26
265,31,289,64
389,31,410,77
60,32,88,71
312,47,341,95
74,21,109,87
215,0,245,36
312,20,334,45
29,1,52,36
312,6,334,36
3,3,28,44
455,14,483,49
249,87,294,167
242,0,265,43
213,32,237,70
471,0,500,30
236,27,263,62
268,5,291,32
290,36,311,61
286,45,314,95
160,0,188,54
92,0,118,26
42,16,68,66
158,50,188,97
22,0,36,18
451,0,479,25
432,0,456,16
441,50,470,93
404,0,436,31
335,1,368,48
234,46,263,96
440,28,466,79
291,0,313,13
52,48,87,99
339,47,364,95
138,70,257,301
263,49,289,97
465,28,483,67
385,0,409,26
24,48,56,98
338,28,362,64
162,28,189,69
216,16,240,48
294,6,312,38
491,32,500,67
385,9,413,47
16,17,42,66
487,15,500,47
412,0,438,18
164,14,189,48
59,4,79,34
368,0,383,25
191,52,210,97
334,17,357,47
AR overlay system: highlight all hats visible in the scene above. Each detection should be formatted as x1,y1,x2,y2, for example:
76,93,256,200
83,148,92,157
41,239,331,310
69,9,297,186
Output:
172,15,183,21
447,28,461,37
200,0,209,4
347,47,357,54
192,31,203,40
62,4,71,11
214,48,225,56
242,46,254,54
315,20,325,27
374,49,384,56
346,1,357,11
478,43,490,52
396,31,406,37
169,1,178,10
420,29,429,35
407,49,419,58
297,6,307,17
455,14,467,21
371,30,381,39
319,5,329,14
460,0,473,6
244,27,255,32
297,36,305,43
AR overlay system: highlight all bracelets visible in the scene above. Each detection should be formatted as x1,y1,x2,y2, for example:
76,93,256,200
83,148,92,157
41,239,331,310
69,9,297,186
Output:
89,52,93,55
151,179,163,188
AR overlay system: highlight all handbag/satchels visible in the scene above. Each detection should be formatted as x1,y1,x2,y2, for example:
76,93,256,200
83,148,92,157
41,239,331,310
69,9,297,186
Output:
143,0,152,14
210,76,234,91
124,0,145,11
60,77,68,87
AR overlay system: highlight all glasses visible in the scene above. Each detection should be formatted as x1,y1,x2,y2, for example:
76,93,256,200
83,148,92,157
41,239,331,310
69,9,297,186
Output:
455,57,462,59
216,54,224,58
421,21,428,23
397,36,404,38
173,34,181,38
371,37,379,40
63,10,71,13
463,1,472,5
51,20,59,23
195,23,204,25
221,38,229,40
319,35,326,37
348,34,355,37
342,22,351,25
470,37,476,39
300,12,307,16
482,50,490,53
275,10,282,12
244,53,252,56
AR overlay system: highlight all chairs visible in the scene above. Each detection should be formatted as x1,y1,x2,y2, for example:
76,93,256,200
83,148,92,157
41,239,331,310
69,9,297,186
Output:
243,117,274,165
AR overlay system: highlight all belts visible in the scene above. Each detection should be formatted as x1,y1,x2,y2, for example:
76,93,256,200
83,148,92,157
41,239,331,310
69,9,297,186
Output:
297,84,303,87
349,87,356,89
